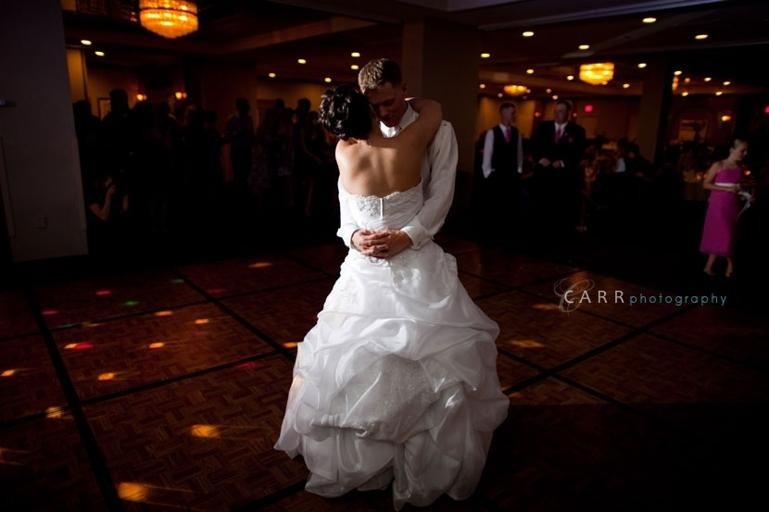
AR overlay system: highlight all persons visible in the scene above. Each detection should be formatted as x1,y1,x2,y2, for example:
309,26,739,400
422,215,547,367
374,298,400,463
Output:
68,86,769,281
334,55,461,260
316,86,457,511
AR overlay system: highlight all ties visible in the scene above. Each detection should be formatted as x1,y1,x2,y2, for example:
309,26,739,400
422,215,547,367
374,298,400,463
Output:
555,126,562,145
504,127,511,143
